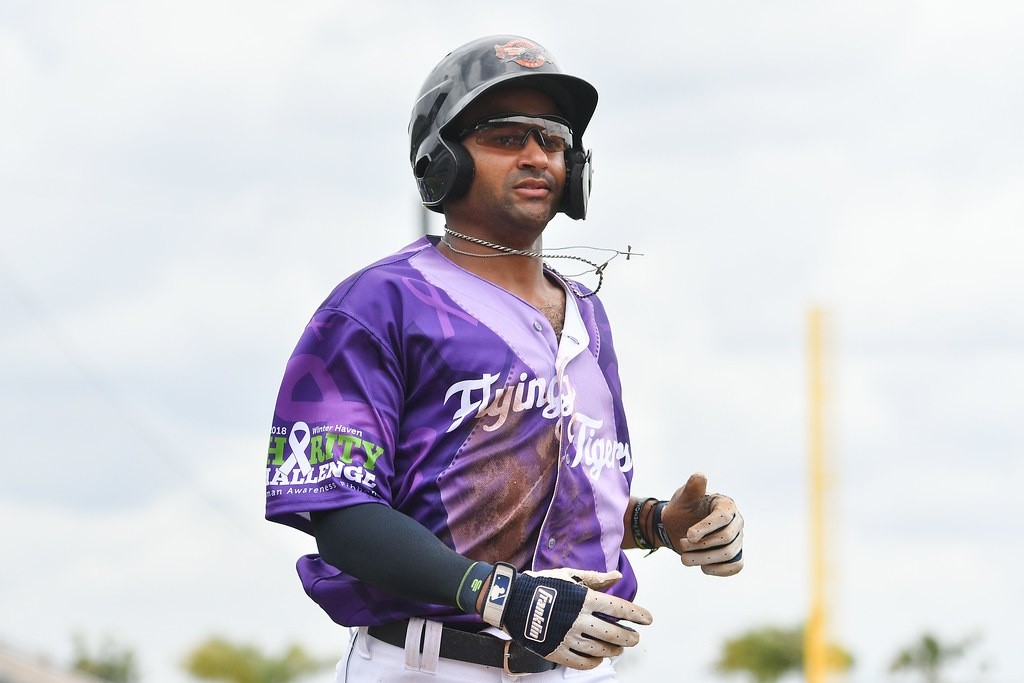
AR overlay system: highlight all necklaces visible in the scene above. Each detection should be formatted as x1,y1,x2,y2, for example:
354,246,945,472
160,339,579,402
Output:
441,224,644,299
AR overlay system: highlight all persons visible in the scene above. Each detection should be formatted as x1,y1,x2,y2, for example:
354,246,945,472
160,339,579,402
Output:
265,34,744,683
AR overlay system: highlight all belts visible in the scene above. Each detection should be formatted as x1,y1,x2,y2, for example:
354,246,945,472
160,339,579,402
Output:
366,611,558,677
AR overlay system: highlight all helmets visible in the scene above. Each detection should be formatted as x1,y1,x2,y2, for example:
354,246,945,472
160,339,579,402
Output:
408,35,598,222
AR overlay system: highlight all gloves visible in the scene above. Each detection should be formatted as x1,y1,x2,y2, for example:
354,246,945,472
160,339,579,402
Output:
653,473,743,576
482,561,653,671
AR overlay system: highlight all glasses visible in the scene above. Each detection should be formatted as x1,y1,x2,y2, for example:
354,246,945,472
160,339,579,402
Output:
454,112,574,152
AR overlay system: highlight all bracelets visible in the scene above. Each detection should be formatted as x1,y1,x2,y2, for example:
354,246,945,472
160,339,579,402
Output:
630,497,674,559
